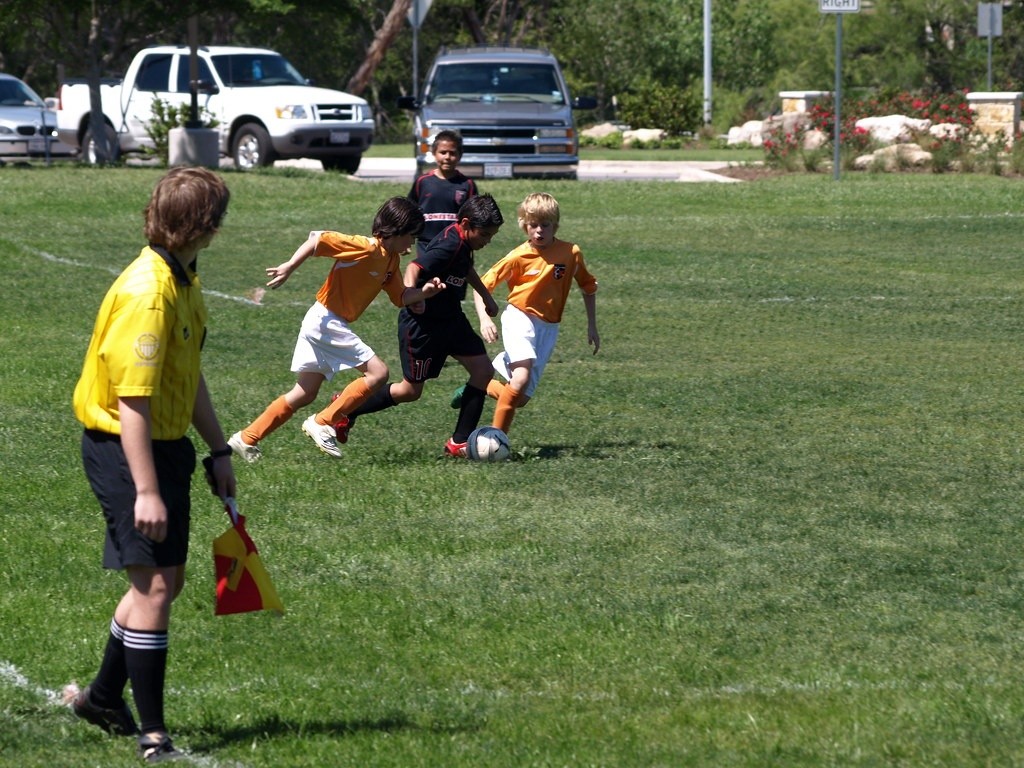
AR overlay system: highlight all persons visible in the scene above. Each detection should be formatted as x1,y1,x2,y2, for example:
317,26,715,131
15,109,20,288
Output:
407,129,479,267
332,192,505,459
450,193,600,435
73,166,237,766
227,195,446,463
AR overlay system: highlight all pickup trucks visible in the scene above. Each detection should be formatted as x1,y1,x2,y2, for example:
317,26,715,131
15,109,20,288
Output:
52,44,378,179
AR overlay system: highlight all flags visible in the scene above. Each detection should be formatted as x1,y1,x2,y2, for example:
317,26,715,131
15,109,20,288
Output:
212,498,287,616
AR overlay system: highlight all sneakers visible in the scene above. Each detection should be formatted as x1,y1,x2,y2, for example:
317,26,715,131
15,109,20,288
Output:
140,736,185,762
74,685,141,737
331,393,356,444
301,414,344,459
449,384,467,409
226,430,263,465
444,436,469,459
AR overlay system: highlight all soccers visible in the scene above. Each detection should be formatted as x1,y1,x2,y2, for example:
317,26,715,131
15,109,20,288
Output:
464,425,512,465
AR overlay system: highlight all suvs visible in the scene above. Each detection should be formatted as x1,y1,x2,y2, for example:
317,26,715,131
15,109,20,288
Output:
395,45,600,184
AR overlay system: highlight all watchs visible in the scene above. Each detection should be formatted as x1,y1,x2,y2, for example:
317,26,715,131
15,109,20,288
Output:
208,444,233,456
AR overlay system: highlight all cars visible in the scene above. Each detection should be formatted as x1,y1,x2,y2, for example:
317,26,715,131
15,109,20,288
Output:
0,73,58,163
